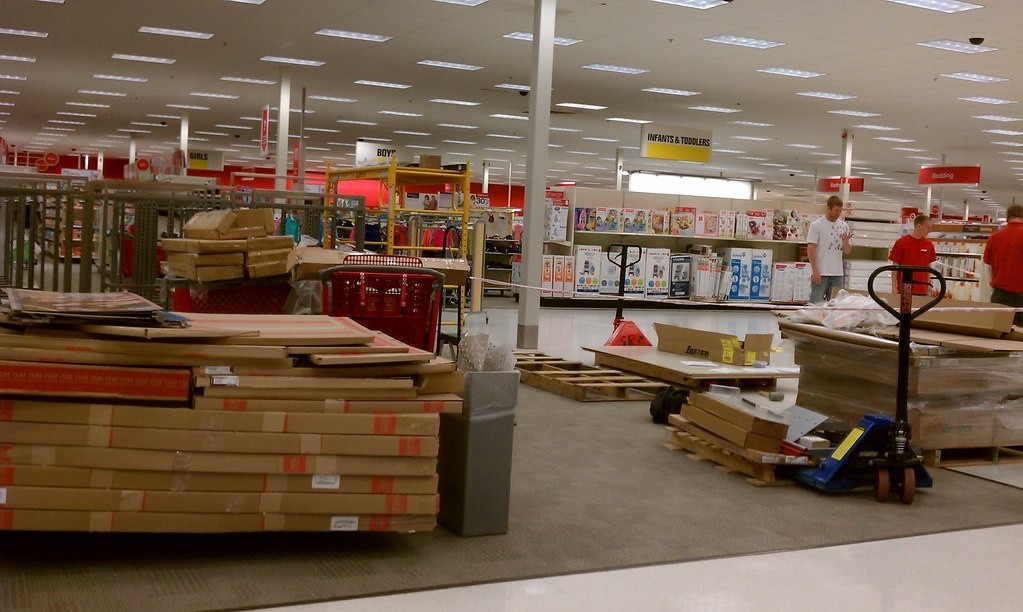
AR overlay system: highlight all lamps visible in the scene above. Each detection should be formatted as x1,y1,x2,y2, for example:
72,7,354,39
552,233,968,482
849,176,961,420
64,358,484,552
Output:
623,169,762,201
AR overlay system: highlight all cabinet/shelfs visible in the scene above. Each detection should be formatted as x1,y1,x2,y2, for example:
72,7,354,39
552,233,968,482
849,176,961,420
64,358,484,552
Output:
469,207,519,297
58,168,103,259
927,224,1000,283
36,198,56,259
514,187,826,310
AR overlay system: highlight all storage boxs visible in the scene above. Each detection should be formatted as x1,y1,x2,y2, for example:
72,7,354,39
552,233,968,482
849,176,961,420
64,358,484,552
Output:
0,398,440,435
652,322,1023,464
0,273,457,389
0,485,440,517
202,388,418,400
182,207,275,241
0,464,439,495
160,261,244,283
160,238,248,254
245,260,287,280
0,509,437,532
166,252,245,267
245,249,289,266
247,236,295,252
0,361,190,401
192,394,463,414
0,421,439,458
0,443,437,476
402,191,820,305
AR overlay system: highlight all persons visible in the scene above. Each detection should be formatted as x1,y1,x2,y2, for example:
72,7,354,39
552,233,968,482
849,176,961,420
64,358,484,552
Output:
807,195,855,304
983,205,1023,327
888,215,936,296
423,195,437,210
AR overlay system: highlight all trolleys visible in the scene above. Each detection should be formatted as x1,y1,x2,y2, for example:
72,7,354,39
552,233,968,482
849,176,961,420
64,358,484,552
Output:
606,244,651,347
320,253,446,356
775,265,946,504
442,226,462,304
105,228,167,293
166,271,296,315
